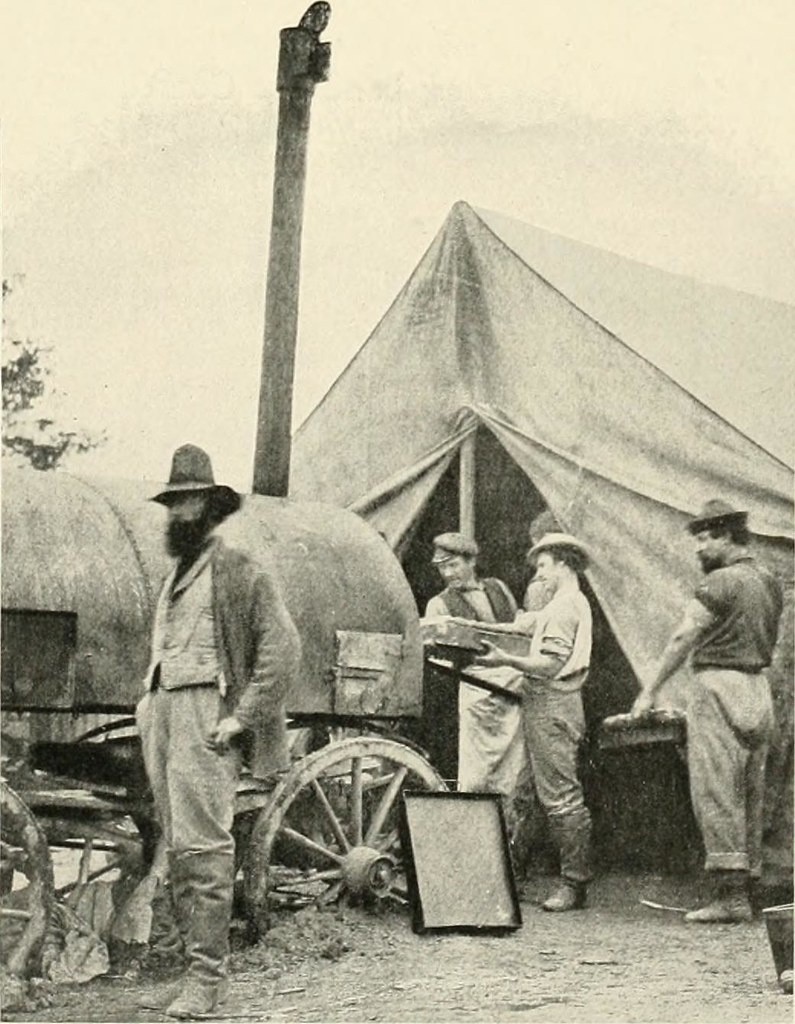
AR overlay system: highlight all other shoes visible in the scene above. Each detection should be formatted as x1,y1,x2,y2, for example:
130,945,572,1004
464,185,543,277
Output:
543,885,586,910
683,894,751,925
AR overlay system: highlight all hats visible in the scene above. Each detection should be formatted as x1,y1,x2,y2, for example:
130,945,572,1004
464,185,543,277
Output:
527,533,590,573
151,443,240,515
431,532,480,564
687,499,749,536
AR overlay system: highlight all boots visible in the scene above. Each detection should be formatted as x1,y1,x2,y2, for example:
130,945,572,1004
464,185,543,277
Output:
138,850,234,1016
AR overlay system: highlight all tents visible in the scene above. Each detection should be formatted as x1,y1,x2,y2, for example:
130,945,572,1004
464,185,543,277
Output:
289,200,795,886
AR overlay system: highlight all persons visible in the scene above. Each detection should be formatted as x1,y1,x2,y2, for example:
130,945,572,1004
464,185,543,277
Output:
130,445,304,1016
633,499,785,922
407,509,598,909
